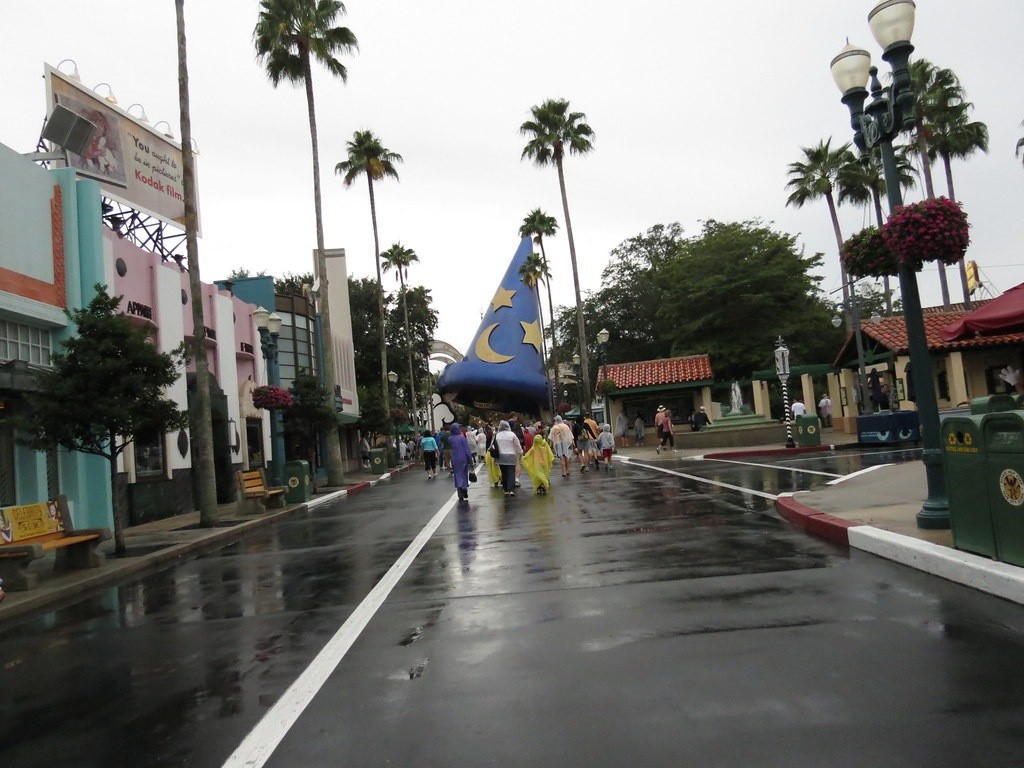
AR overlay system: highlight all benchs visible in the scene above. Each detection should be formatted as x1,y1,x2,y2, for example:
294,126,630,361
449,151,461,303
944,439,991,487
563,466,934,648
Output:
0,494,113,591
234,467,289,515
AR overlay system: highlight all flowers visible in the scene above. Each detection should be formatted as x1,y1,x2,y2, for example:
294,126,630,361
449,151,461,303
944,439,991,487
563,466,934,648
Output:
879,195,972,272
839,225,900,279
252,385,293,410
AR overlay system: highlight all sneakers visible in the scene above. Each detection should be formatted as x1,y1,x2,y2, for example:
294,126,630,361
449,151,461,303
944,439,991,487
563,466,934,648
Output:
536,488,546,494
656,447,662,454
428,474,432,478
670,450,678,454
433,472,438,477
503,489,509,494
509,491,516,496
514,480,521,487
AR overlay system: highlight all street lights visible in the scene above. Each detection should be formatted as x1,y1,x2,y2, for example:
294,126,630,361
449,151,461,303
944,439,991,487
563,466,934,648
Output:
387,370,401,465
831,299,881,416
831,0,951,529
596,327,610,434
251,305,289,486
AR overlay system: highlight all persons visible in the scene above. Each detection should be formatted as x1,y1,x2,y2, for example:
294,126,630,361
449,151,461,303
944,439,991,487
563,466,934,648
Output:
792,399,806,420
655,405,678,454
689,406,711,432
48,502,63,531
0,515,13,542
417,413,614,500
880,384,891,410
634,411,644,446
361,433,370,468
393,439,415,460
616,411,630,447
819,394,832,429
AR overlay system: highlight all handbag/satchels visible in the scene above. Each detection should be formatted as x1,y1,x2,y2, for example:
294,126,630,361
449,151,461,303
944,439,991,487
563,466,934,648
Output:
488,434,499,459
469,465,477,482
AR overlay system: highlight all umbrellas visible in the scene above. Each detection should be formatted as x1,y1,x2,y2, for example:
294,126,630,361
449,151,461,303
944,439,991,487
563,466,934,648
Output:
939,283,1024,343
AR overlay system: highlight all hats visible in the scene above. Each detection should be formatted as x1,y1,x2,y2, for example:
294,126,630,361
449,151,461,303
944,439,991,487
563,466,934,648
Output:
656,405,666,411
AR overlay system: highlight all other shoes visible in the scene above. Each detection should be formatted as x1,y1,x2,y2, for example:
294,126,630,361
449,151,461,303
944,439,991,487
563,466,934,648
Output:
463,494,468,498
440,467,443,470
567,472,570,476
460,498,463,501
580,465,586,472
562,474,566,477
363,464,372,469
494,482,499,487
585,467,591,472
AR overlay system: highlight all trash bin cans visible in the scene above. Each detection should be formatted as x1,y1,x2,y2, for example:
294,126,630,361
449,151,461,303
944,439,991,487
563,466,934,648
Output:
371,447,388,475
969,394,1024,415
795,413,820,448
284,459,311,504
939,410,1024,569
